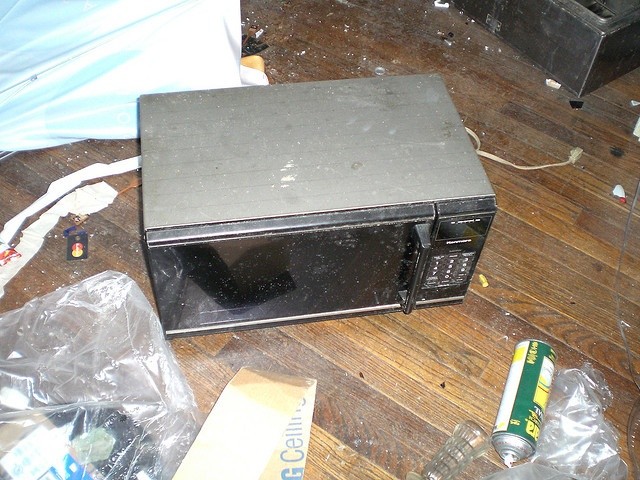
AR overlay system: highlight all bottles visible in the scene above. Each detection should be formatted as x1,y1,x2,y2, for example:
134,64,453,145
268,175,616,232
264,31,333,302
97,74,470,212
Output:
490,338,559,468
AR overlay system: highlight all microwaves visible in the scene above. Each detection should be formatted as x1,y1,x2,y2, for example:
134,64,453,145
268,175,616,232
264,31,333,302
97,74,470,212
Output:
139,72,497,339
